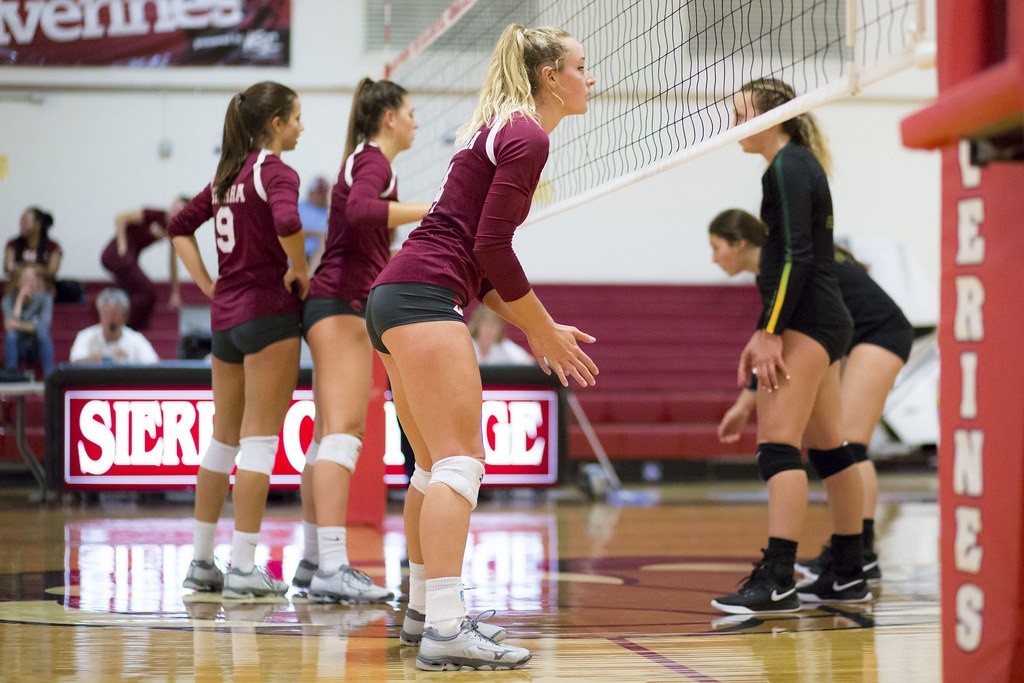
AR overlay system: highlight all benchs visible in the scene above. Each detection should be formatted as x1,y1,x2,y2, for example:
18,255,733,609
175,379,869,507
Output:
0,282,807,469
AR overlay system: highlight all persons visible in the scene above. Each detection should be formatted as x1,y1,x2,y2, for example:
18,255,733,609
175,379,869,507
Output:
710,76,913,614
168,23,599,671
0,196,192,504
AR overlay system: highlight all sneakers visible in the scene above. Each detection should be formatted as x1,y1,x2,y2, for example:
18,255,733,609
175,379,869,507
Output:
799,606,873,630
793,541,883,581
399,606,508,646
307,565,394,604
798,572,872,606
222,565,288,598
415,609,532,670
183,592,252,621
711,568,805,615
292,555,316,588
183,560,225,590
711,615,800,636
221,598,290,635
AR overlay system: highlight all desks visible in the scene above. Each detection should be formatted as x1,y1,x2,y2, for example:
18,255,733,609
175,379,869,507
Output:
0,381,48,503
